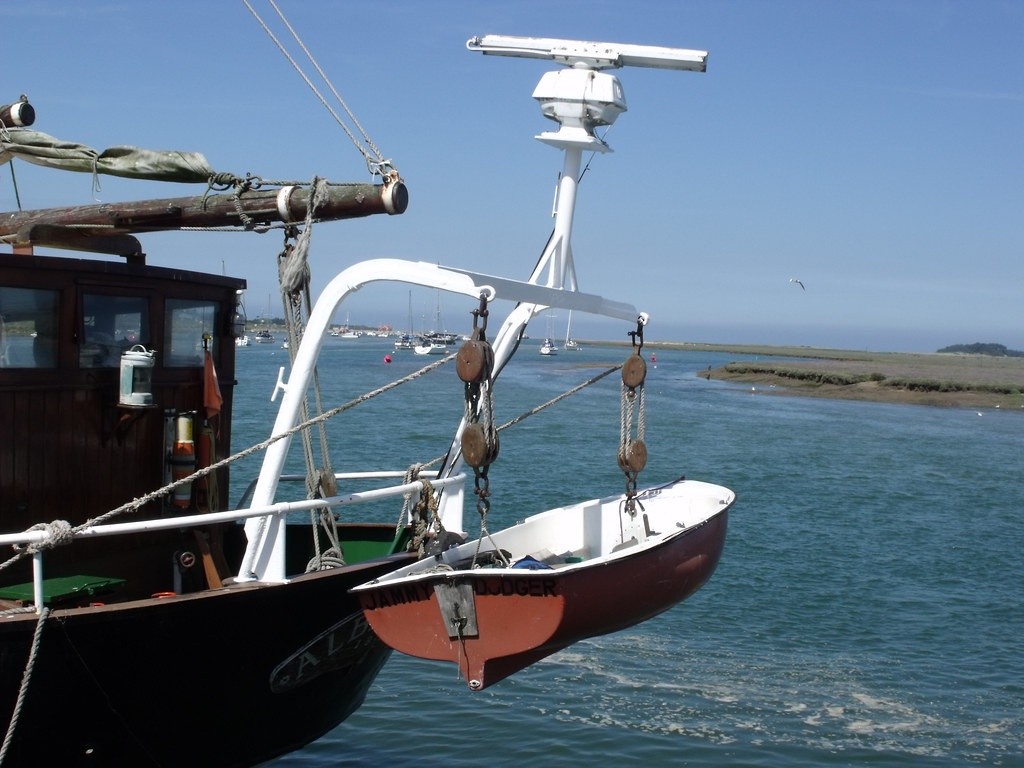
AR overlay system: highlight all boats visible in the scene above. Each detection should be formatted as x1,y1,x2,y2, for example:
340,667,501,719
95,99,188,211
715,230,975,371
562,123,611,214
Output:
1,2,736,768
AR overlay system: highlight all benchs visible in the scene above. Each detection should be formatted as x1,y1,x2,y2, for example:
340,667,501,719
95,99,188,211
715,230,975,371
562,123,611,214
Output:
0,555,161,602
